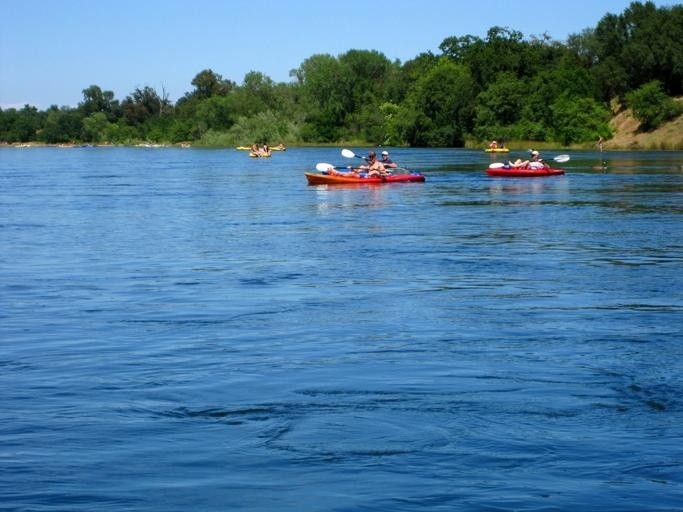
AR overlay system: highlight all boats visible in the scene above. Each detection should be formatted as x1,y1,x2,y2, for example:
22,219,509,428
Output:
484,148,510,154
303,170,427,187
235,146,287,158
485,168,566,177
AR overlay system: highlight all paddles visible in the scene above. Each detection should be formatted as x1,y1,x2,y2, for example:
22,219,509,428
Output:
341,149,387,165
488,154,570,169
316,162,386,172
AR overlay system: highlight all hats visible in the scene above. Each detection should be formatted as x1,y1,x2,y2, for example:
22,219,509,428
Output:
382,151,388,156
532,151,538,155
368,152,376,156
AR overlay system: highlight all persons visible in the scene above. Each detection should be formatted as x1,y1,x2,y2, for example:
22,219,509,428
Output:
490,141,497,149
598,135,604,153
262,144,268,153
327,151,386,179
251,141,263,158
508,151,549,170
365,151,398,168
